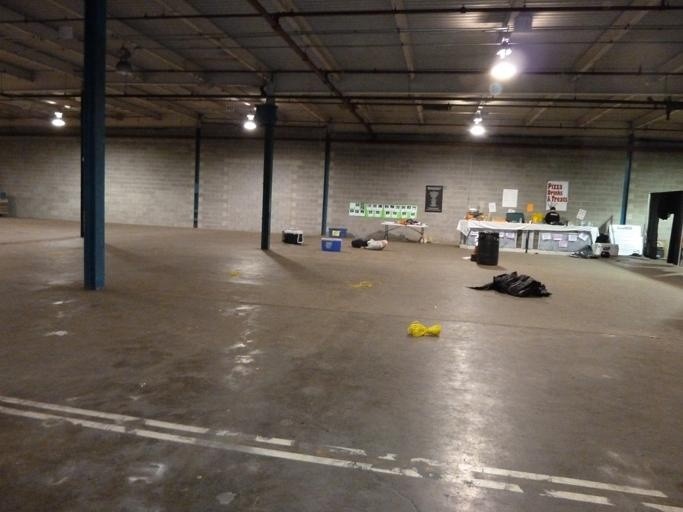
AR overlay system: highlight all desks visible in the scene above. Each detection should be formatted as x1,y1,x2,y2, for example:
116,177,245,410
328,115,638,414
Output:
456,219,600,258
382,221,427,243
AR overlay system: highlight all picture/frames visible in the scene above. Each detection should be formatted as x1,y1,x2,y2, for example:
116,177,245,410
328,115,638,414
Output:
425,185,442,212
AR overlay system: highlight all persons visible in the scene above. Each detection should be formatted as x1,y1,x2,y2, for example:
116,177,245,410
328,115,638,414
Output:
544,207,560,225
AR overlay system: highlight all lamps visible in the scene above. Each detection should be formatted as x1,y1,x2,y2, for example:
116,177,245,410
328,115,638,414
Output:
244,109,256,131
489,31,516,80
466,108,485,136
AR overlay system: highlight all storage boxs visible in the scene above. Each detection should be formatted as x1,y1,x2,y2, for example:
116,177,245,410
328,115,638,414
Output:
282,230,304,244
320,237,342,253
329,228,346,237
